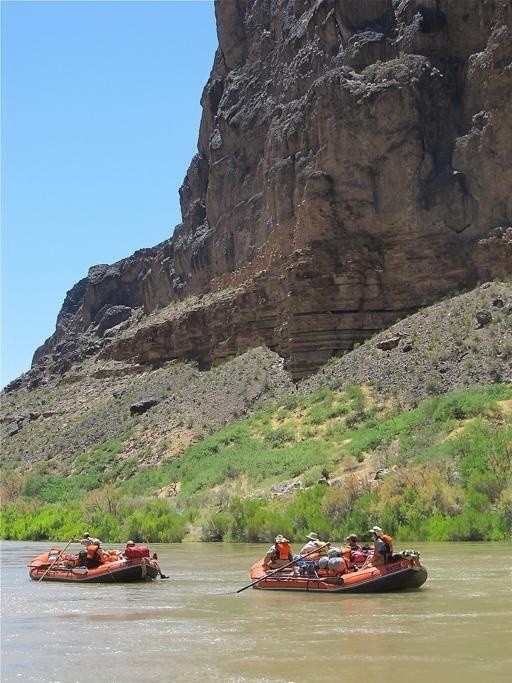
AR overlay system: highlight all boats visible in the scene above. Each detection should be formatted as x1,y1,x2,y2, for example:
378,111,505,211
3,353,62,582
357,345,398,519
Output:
249,547,428,592
27,547,161,581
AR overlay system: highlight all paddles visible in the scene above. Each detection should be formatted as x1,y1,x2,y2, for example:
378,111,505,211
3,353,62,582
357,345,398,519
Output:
27,565,88,575
252,577,345,585
237,543,328,593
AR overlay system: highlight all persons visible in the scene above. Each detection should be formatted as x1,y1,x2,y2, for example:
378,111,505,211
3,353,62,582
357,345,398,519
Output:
48,532,169,579
262,526,393,577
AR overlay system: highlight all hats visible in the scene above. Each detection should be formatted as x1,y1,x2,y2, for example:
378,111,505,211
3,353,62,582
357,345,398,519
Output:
305,532,317,541
276,535,290,544
368,526,384,539
83,532,90,539
346,534,358,542
93,539,102,545
